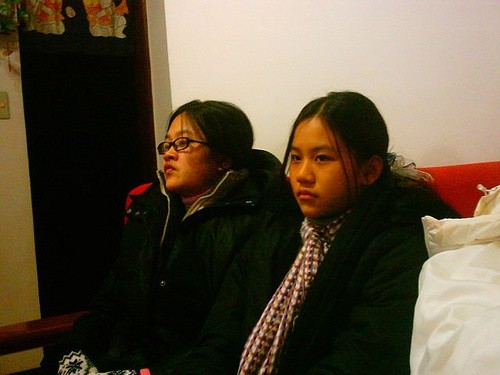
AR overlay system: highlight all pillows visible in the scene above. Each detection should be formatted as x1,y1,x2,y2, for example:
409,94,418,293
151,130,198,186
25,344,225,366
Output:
410,241,500,375
422,214,500,258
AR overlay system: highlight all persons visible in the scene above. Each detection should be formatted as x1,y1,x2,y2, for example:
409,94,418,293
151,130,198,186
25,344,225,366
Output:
40,101,289,375
234,93,428,375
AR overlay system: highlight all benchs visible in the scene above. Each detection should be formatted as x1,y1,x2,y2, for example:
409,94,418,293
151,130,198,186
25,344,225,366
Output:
122,161,500,218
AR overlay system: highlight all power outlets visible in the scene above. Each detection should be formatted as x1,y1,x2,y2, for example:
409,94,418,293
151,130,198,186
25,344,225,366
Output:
0,91,10,118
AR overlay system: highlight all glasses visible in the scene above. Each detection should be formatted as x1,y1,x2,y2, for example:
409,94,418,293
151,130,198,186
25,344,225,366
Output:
157,137,214,155
